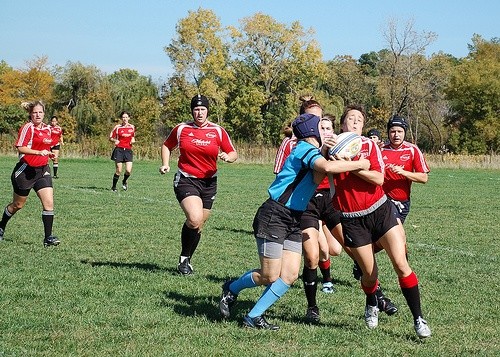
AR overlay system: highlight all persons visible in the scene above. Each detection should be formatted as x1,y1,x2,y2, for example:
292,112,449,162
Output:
158,94,238,274
109,111,135,191
219,94,431,340
46,116,65,178
0,99,60,246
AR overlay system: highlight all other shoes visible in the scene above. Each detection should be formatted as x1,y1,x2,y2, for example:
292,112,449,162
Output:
43,237,61,247
111,186,118,192
0,221,6,239
53,174,59,179
122,179,128,190
322,281,334,294
352,260,361,280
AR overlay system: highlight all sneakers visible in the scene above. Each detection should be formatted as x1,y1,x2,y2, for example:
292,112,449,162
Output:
237,315,280,331
219,280,238,321
376,293,398,316
413,317,432,337
178,255,193,276
364,304,379,329
304,305,320,323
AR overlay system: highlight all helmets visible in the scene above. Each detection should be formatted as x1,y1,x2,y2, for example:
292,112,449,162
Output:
190,94,208,109
292,114,321,140
385,115,409,130
367,128,380,137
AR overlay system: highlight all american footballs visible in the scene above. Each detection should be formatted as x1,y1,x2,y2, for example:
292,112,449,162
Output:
329,132,362,159
